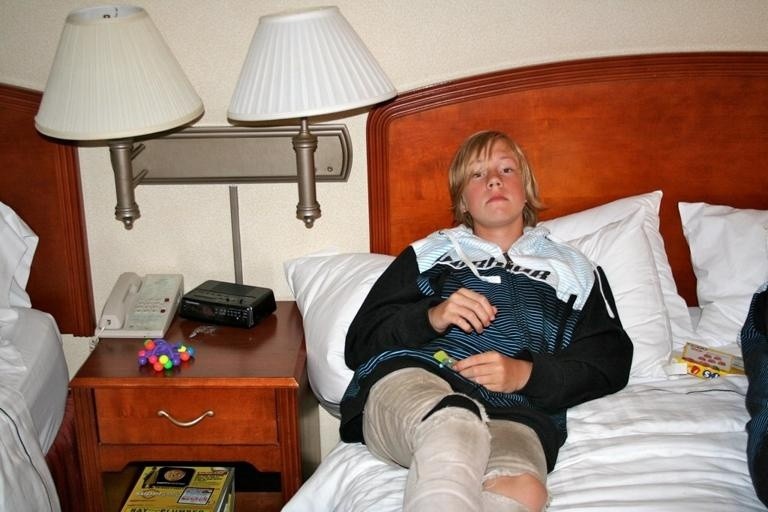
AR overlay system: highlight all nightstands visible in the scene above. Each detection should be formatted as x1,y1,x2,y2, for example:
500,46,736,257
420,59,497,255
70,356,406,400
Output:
69,301,320,512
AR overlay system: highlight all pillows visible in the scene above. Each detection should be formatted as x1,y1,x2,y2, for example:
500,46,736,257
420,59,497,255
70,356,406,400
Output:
677,201,767,348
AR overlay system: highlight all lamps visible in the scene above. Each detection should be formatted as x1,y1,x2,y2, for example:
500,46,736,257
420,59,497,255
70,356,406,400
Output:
34,5,397,228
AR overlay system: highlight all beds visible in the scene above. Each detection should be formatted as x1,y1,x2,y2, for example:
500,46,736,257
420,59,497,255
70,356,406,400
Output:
356,52,768,512
0,83,97,512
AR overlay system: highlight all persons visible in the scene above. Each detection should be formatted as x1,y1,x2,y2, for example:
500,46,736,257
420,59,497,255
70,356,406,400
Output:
337,126,634,510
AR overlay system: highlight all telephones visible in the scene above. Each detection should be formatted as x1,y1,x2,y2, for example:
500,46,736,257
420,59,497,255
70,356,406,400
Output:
94,272,183,339
